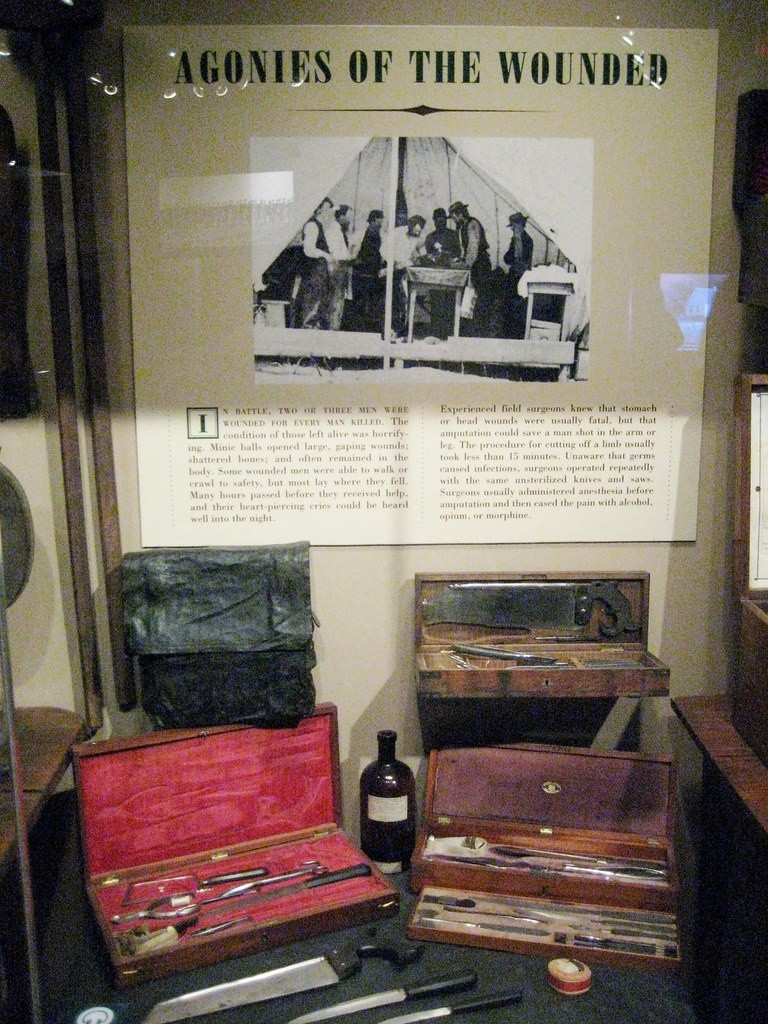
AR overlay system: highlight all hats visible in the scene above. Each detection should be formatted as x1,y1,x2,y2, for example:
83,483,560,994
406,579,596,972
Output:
445,201,468,220
506,212,529,227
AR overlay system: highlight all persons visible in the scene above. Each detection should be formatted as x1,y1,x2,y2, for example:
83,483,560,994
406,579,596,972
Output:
295,195,535,336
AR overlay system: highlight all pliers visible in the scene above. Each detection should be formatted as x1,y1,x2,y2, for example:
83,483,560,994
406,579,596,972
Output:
448,642,559,666
108,890,195,924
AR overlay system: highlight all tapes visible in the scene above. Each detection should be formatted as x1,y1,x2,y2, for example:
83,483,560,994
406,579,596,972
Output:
546,956,593,996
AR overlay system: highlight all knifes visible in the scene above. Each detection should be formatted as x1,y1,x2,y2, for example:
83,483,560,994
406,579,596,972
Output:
200,863,372,918
143,938,425,1024
379,990,523,1024
288,969,478,1024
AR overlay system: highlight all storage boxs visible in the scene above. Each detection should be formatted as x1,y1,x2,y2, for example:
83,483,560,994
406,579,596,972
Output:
71,703,400,986
409,733,682,971
414,570,670,699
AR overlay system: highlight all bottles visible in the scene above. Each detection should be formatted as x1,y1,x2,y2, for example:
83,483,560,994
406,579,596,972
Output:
360,729,417,876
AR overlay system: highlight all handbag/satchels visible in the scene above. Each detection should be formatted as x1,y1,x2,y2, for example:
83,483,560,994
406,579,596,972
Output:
123,538,321,729
